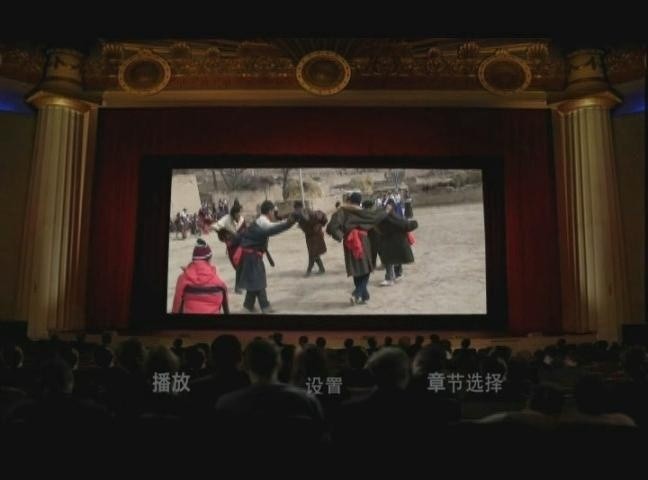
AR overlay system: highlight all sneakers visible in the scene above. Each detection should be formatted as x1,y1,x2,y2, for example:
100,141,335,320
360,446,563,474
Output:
395,274,403,281
349,295,368,306
379,279,393,285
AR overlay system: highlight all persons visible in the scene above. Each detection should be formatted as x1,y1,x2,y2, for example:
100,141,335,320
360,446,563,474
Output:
170,188,417,315
0,328,647,479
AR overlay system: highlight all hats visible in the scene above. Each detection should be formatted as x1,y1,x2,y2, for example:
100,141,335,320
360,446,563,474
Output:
191,238,212,260
